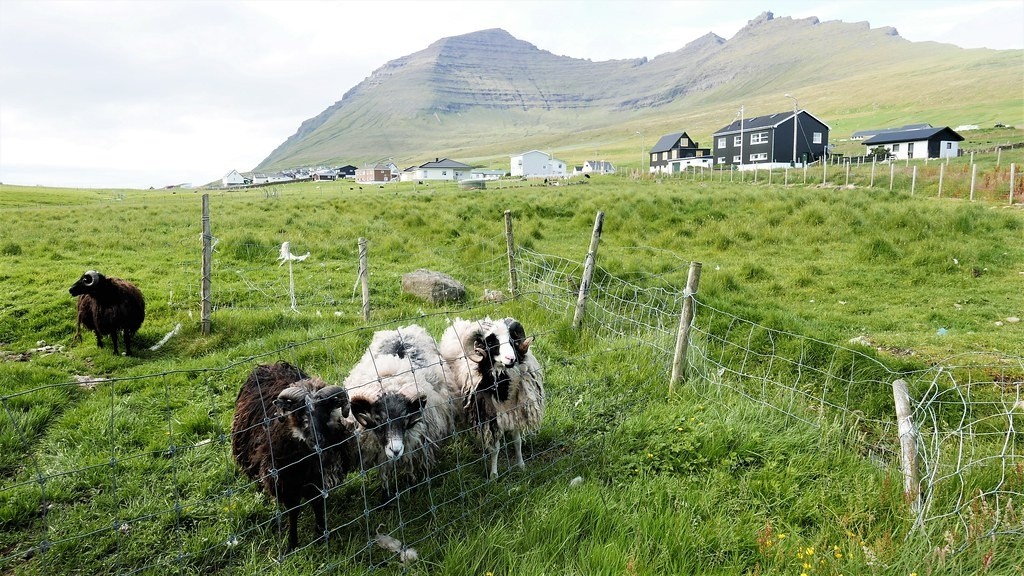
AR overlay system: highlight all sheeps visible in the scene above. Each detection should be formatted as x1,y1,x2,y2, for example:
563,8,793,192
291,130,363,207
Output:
66,270,148,357
230,318,553,548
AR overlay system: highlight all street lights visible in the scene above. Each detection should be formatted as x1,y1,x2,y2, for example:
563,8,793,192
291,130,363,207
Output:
636,131,645,180
783,93,798,163
